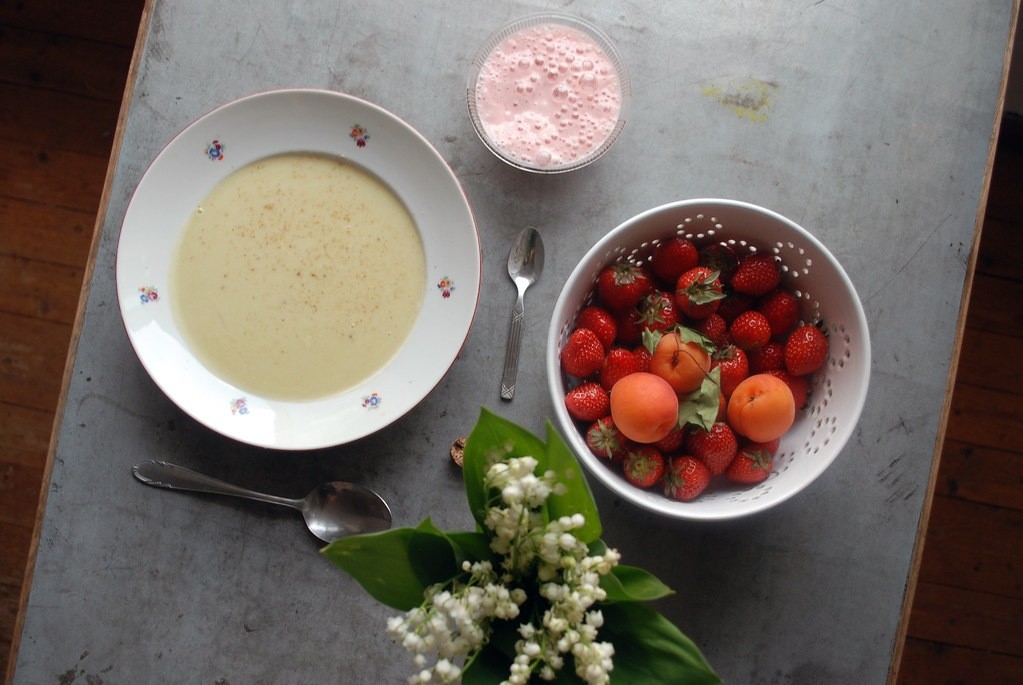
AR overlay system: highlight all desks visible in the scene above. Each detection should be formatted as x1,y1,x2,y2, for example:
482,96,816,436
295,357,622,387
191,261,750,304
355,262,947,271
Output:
3,0,1022,685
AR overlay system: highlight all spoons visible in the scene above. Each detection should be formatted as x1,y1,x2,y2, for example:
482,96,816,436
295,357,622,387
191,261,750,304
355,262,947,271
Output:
501,226,545,401
132,460,393,544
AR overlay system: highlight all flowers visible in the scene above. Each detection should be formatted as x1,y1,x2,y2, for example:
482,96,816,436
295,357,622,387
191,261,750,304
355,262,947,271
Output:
317,406,724,685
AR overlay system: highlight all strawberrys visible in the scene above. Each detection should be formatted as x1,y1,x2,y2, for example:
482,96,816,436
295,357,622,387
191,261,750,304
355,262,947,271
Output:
559,237,831,501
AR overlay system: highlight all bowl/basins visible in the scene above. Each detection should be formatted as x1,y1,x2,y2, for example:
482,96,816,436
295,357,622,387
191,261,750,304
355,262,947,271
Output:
466,11,633,175
545,198,871,523
115,88,482,452
